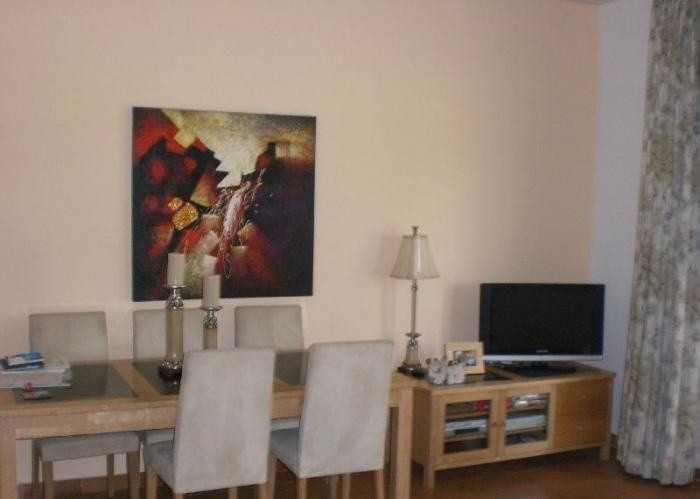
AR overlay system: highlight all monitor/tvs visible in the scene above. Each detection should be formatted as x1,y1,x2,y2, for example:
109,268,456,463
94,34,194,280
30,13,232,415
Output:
477,282,606,380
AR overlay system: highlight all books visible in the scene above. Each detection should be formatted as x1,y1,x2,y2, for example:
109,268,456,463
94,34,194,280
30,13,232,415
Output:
462,393,550,412
0,353,73,390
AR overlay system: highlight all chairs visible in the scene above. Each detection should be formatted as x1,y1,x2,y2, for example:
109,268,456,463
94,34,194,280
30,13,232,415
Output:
28,312,141,499
132,307,207,445
142,349,276,499
268,339,393,499
236,305,339,498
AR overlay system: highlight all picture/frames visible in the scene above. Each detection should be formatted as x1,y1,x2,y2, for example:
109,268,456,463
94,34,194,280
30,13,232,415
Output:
444,342,484,374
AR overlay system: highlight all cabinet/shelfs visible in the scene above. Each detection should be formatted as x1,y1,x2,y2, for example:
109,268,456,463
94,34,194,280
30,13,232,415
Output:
411,360,616,490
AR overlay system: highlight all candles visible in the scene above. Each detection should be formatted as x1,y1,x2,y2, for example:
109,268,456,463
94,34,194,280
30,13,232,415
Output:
203,275,220,308
168,253,185,286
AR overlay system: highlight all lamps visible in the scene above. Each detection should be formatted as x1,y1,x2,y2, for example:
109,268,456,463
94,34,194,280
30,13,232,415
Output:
390,226,439,375
